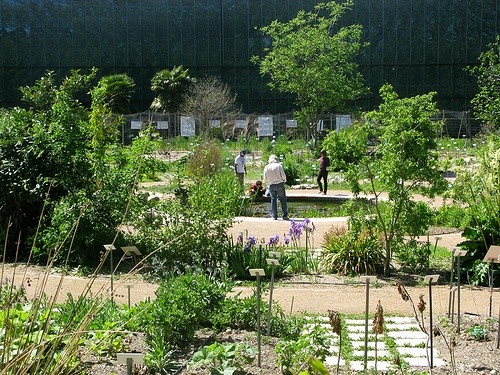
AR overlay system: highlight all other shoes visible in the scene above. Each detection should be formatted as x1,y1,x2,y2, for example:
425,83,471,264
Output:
283,216,288,220
324,193,326,195
318,191,322,194
274,216,277,220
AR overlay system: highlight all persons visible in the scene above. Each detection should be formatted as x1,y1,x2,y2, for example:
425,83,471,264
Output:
235,149,247,196
247,180,266,197
317,150,330,195
264,154,290,220
